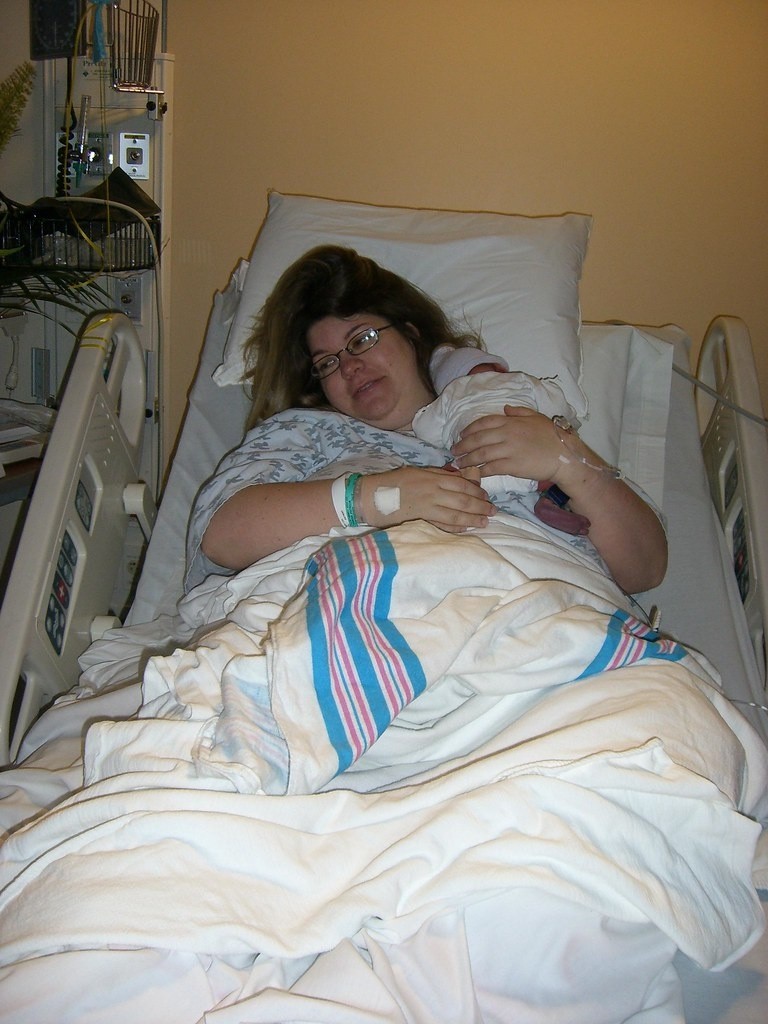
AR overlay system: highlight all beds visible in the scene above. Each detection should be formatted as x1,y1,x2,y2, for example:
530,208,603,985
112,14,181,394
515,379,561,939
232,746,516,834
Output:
0,313,767,1024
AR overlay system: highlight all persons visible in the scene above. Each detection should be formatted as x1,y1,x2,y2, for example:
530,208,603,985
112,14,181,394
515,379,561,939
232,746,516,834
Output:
411,347,591,536
183,245,668,597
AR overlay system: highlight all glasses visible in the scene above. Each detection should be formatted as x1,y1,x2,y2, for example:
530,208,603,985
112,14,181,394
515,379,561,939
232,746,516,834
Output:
308,322,393,385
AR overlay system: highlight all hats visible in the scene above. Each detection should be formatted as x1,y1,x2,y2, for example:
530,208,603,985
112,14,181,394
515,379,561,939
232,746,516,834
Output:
430,342,511,396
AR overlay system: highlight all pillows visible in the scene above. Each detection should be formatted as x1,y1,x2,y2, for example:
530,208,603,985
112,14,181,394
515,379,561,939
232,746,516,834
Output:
575,322,674,505
210,188,595,421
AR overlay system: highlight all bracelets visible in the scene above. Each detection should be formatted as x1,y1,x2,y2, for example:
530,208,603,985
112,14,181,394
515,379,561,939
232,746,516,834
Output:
331,471,363,529
